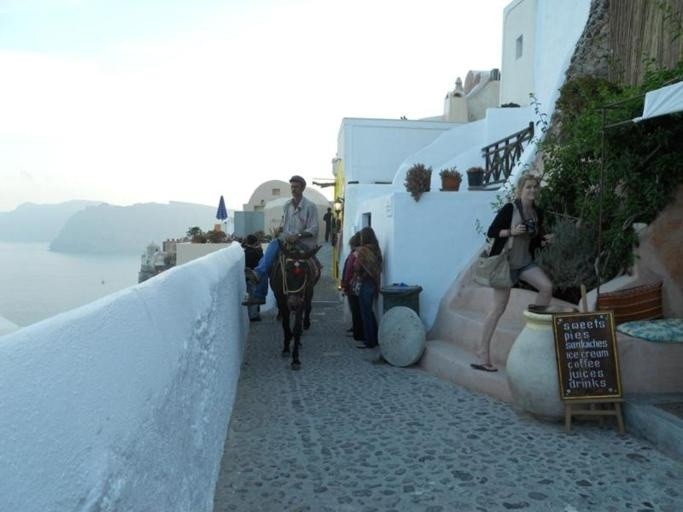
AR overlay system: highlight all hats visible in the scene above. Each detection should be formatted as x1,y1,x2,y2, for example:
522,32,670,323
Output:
289,176,306,186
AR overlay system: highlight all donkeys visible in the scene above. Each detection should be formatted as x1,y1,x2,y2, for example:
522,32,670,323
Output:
270,239,322,370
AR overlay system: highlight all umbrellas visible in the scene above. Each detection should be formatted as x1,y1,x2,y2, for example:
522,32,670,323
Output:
215,195,227,224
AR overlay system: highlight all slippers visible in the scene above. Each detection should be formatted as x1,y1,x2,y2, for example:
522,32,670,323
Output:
470,359,498,372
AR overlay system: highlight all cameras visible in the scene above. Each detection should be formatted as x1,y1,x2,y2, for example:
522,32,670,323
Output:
524,222,536,237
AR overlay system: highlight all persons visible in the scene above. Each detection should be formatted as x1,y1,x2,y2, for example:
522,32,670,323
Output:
243,235,265,323
351,227,383,348
240,175,319,306
339,232,363,342
467,175,554,371
322,206,332,241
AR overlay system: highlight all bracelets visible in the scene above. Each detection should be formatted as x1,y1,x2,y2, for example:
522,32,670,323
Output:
506,228,511,236
297,233,303,238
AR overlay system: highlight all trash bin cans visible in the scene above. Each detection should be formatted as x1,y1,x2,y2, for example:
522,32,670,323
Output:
379,282,423,317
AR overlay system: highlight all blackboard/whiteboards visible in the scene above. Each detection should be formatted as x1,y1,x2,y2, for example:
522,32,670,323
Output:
552,310,622,400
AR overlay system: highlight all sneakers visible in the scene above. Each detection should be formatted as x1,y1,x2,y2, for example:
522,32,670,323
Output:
242,296,266,305
244,269,260,285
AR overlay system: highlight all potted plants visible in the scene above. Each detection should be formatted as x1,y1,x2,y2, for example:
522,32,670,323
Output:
404,164,484,202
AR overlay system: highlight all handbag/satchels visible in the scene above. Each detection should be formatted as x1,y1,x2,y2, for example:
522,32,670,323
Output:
472,249,515,289
